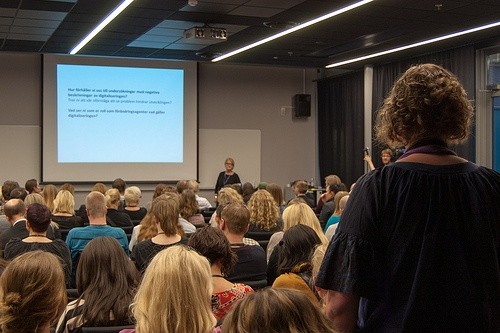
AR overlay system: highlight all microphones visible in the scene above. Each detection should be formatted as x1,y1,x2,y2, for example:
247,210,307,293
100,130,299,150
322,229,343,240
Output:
361,147,369,151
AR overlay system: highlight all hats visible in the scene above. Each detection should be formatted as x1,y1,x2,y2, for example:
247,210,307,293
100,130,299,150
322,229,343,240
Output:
258,183,267,189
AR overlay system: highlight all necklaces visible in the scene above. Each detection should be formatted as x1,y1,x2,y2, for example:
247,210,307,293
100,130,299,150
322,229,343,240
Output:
230,243,242,245
29,234,45,237
58,211,68,213
158,232,176,234
223,170,233,185
126,205,138,207
212,275,222,277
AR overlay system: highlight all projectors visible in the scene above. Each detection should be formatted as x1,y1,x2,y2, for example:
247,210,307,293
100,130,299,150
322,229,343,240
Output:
183,26,227,40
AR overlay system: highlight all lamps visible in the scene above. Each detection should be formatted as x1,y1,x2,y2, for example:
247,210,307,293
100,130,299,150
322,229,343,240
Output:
67,0,134,55
325,12,500,68
199,0,374,63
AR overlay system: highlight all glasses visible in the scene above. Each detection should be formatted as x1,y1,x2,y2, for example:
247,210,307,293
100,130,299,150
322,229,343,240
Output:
225,162,234,165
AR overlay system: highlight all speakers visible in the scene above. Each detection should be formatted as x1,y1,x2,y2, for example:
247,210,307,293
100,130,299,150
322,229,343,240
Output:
292,93,311,118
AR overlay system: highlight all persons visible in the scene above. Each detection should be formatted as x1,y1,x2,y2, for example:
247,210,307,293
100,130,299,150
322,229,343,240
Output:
0,149,395,333
316,64,500,333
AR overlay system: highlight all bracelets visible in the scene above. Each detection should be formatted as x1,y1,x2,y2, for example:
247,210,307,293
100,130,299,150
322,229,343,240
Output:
215,195,217,199
